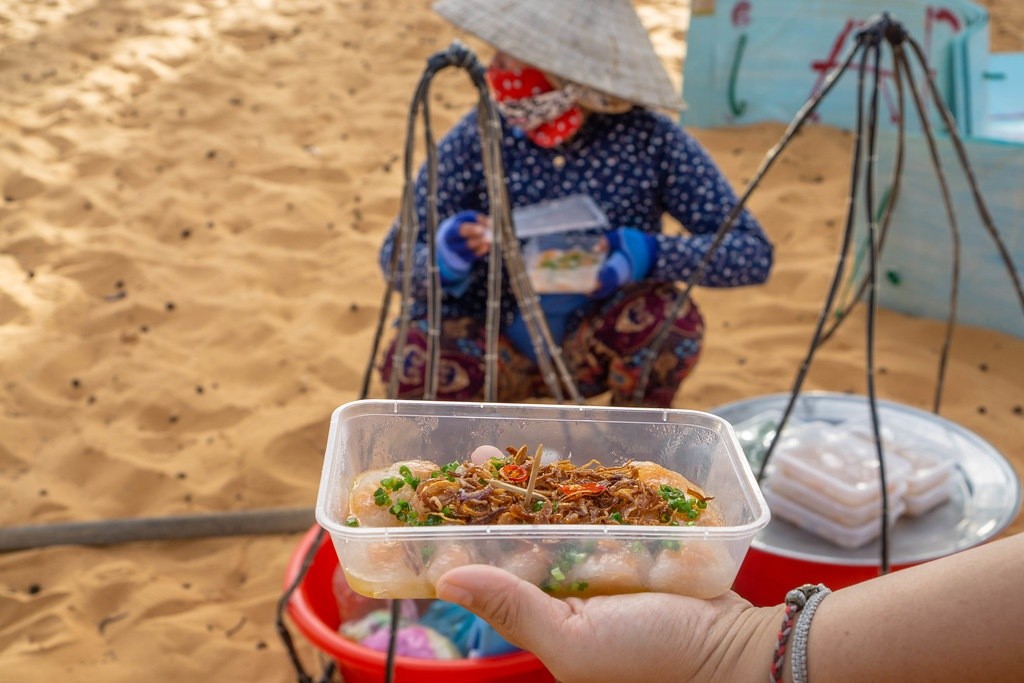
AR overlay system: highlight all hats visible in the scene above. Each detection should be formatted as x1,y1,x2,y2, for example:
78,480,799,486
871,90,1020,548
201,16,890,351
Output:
431,1,688,112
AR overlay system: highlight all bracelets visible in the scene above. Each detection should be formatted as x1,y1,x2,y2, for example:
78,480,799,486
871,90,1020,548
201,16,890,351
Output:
769,582,834,683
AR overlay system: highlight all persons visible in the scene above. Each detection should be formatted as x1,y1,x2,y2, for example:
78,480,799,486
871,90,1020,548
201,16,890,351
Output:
378,2,775,464
434,532,1024,683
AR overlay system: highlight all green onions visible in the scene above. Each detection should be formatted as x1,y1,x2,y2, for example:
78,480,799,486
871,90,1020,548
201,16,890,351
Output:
347,459,708,593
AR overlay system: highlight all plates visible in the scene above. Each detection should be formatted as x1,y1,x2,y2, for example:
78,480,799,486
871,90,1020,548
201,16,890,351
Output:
689,390,1019,567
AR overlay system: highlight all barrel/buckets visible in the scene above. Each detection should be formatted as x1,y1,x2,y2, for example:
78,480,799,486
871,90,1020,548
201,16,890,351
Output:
281,521,562,683
728,549,927,608
